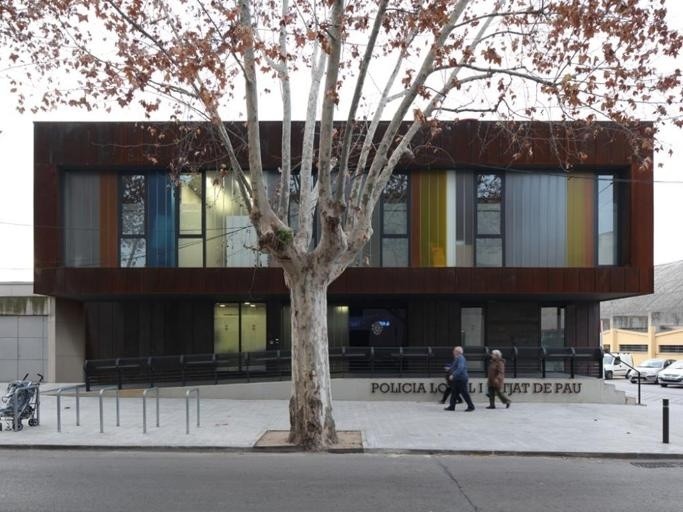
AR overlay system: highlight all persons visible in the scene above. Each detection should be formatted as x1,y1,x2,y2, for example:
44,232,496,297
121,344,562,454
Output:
442,346,475,411
437,350,463,404
485,349,512,409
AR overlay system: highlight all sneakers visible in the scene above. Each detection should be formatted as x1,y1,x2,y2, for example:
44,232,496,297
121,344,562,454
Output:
438,399,513,413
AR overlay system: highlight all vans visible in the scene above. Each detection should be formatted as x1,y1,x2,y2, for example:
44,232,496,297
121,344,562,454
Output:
602,352,633,380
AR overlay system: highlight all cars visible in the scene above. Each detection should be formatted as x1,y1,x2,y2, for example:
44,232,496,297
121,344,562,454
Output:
627,351,683,388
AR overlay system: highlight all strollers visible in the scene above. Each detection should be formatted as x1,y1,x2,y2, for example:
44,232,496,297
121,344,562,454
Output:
0,373,43,431
